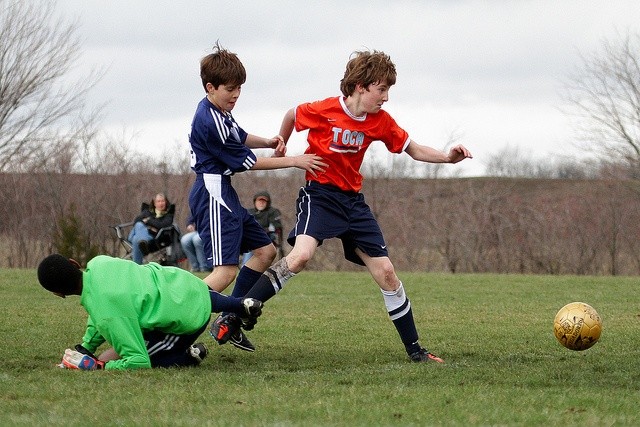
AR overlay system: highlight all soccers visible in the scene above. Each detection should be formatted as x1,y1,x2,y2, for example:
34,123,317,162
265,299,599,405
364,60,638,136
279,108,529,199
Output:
553,302,603,351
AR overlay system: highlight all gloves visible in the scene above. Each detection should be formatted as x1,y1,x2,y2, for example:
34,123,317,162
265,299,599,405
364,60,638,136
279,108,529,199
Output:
62,343,105,371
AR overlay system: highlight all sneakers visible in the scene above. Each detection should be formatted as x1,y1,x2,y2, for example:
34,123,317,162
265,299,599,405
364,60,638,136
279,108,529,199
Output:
209,312,243,345
139,238,149,255
242,297,264,330
176,342,208,367
411,347,446,364
214,314,255,352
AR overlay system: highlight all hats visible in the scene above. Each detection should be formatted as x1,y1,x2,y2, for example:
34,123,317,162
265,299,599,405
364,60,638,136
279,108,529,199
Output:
257,190,269,202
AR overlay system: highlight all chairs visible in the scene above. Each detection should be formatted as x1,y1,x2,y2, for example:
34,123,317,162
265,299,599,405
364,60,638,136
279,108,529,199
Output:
109,202,182,268
253,208,285,264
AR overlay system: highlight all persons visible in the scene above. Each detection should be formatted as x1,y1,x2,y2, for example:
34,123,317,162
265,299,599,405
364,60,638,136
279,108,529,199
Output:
127,192,175,264
180,211,209,272
187,38,277,353
37,253,264,372
209,48,474,363
239,191,282,270
